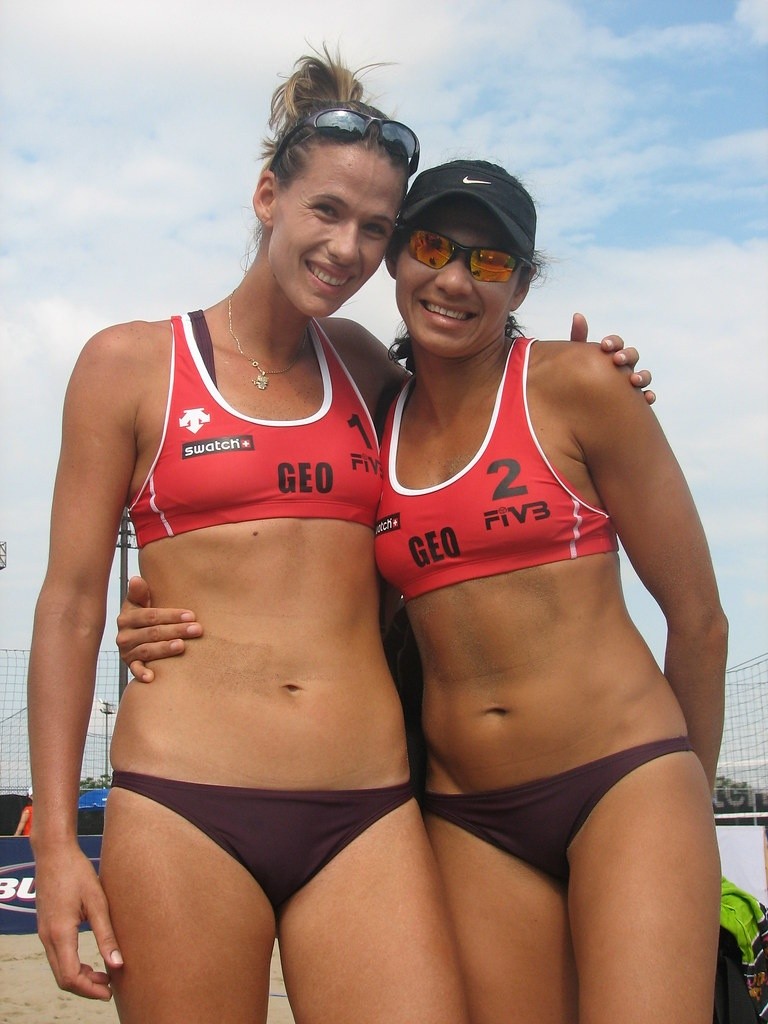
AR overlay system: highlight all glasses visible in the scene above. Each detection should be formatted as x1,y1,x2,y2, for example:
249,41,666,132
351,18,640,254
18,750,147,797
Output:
405,227,522,282
269,107,420,172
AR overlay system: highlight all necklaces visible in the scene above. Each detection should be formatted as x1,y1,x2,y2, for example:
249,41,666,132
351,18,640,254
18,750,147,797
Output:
229,288,308,390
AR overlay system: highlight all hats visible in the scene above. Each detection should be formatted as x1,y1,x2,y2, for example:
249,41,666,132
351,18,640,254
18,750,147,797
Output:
397,164,535,262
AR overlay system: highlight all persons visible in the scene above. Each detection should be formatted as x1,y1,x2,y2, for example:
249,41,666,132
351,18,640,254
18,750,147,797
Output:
14,787,33,835
27,56,654,1024
116,160,728,1024
